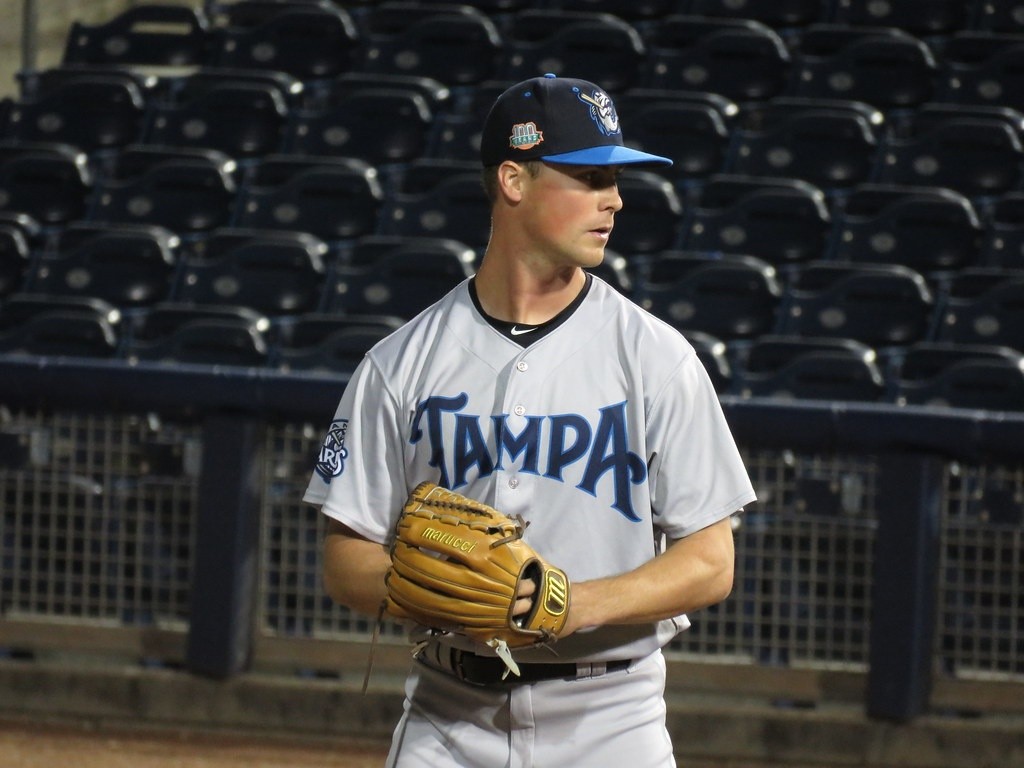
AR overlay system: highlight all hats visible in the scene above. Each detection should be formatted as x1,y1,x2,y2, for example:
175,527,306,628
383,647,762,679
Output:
480,73,673,172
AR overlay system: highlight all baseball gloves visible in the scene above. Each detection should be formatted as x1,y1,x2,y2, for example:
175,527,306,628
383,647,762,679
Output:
387,482,568,647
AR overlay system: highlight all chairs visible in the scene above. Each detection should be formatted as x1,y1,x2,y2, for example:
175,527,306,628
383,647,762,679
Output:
0,0,1024,524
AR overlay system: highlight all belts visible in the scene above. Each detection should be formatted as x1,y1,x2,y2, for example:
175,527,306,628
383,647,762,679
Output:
418,639,640,687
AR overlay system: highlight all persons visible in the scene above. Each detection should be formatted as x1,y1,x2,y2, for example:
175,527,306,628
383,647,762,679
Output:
281,59,760,768
